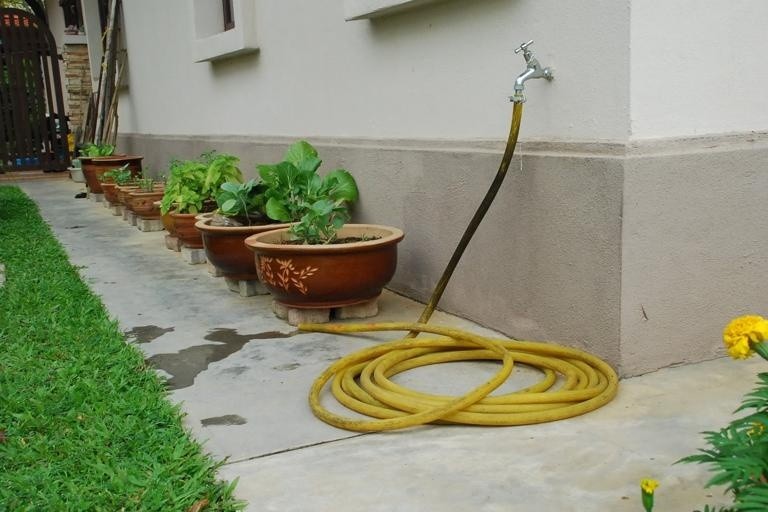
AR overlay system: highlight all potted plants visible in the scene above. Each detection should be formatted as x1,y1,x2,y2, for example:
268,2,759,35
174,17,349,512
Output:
243,161,404,310
79,143,242,249
194,141,322,282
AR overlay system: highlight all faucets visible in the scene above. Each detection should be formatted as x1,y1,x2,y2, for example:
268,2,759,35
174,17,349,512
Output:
508,39,552,105
